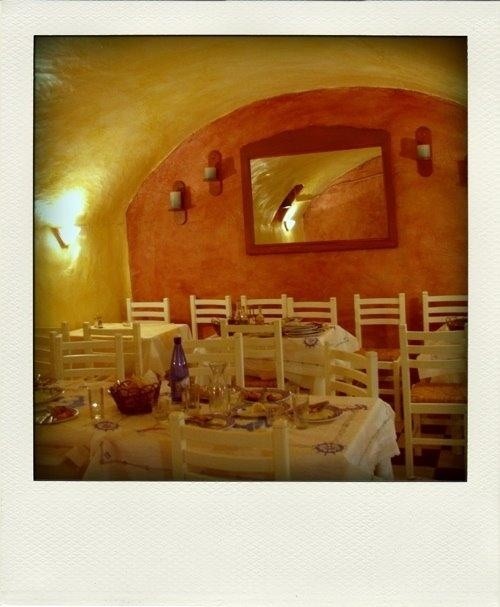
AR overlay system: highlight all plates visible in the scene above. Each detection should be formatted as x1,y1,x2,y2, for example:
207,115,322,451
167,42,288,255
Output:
234,379,343,431
35,404,80,424
266,316,328,338
34,375,57,386
34,385,67,403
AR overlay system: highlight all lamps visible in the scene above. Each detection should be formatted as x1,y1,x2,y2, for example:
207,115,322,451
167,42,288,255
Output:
202,150,223,196
417,125,433,176
167,180,186,226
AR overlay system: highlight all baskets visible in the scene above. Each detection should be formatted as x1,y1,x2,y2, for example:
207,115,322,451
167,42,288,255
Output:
108,372,164,416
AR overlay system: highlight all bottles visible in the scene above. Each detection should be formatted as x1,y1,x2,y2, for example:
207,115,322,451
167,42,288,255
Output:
169,337,191,404
233,298,265,325
207,362,232,418
93,317,102,328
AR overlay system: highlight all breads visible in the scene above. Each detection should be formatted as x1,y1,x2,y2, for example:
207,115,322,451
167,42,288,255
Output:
267,393,281,400
237,391,260,401
207,415,226,426
253,402,267,413
54,405,74,419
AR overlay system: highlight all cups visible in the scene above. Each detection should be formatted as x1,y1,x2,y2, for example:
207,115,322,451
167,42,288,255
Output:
181,383,201,416
87,385,104,425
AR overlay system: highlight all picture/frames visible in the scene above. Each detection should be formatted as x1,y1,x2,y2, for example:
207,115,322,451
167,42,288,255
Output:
239,123,398,256
